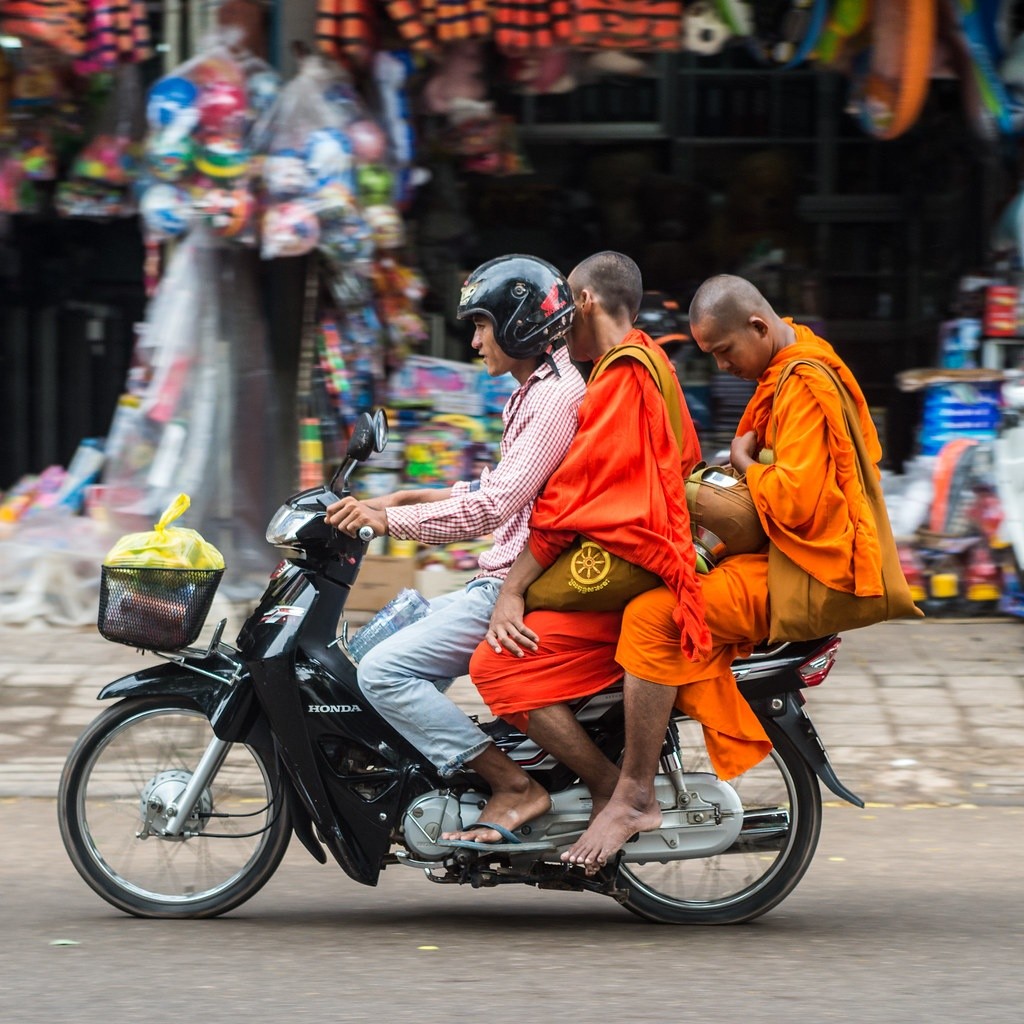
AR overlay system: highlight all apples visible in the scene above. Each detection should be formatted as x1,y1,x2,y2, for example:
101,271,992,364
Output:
107,548,216,587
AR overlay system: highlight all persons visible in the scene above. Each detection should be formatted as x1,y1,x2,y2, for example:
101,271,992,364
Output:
469,252,702,876
323,253,588,853
558,273,924,866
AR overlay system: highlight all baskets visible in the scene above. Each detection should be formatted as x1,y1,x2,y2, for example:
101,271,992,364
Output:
98,565,225,652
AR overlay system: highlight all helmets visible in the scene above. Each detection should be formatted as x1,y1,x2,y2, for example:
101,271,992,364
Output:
457,253,574,358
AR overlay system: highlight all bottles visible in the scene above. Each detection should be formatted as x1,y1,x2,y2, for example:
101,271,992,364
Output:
349,590,430,662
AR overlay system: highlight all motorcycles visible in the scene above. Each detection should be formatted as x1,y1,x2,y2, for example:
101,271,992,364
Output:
54,407,866,928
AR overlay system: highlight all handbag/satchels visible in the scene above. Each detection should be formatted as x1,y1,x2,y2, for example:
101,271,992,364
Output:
685,463,770,552
764,359,925,645
523,344,682,610
105,491,225,589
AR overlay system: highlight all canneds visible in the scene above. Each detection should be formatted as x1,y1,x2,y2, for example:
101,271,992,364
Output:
104,592,188,645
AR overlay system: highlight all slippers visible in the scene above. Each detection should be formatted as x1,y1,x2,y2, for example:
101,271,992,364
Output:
437,822,557,852
